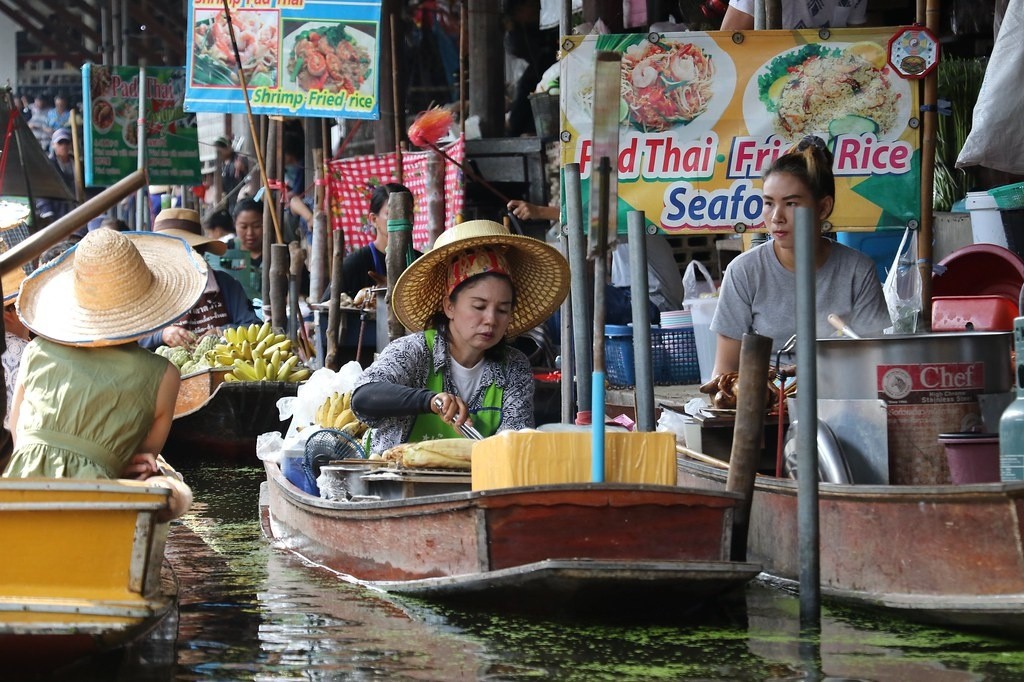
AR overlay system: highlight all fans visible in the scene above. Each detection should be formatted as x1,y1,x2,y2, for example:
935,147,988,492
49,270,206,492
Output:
304,426,367,480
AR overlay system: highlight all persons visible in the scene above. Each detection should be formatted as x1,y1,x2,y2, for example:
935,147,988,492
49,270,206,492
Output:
708,142,891,382
0,229,209,479
290,197,315,249
283,145,304,242
14,94,82,223
507,200,685,343
206,195,264,302
0,239,32,426
721,0,868,31
349,220,572,456
215,137,249,191
321,183,424,301
140,208,260,348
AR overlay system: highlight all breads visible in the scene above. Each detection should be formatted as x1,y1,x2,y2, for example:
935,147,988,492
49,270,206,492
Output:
371,438,479,469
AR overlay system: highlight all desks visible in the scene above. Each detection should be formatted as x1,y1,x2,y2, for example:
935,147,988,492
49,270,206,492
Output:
437,136,549,241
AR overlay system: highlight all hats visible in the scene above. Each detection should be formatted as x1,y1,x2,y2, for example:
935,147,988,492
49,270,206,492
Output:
392,220,571,340
16,226,209,348
154,208,227,255
51,128,72,143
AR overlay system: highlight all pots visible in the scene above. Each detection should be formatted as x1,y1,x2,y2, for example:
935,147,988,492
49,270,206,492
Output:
809,328,1017,487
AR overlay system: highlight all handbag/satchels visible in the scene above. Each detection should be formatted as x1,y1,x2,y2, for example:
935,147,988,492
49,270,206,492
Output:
882,227,923,333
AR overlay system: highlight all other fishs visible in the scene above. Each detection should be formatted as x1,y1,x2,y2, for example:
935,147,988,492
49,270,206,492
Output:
382,438,477,469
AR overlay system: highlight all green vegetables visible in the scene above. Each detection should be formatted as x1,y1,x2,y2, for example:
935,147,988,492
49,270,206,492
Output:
193,53,278,88
758,43,843,112
289,20,371,87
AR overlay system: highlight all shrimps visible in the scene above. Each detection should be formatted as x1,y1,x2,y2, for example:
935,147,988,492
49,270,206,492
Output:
780,57,886,131
626,42,695,88
289,30,359,93
194,8,277,72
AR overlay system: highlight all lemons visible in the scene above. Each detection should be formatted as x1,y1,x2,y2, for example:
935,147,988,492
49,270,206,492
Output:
845,41,888,71
768,75,790,106
619,98,628,122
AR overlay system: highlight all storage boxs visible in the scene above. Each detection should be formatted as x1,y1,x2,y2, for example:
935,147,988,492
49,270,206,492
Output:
605,324,700,386
283,439,320,498
469,429,679,492
931,295,1019,332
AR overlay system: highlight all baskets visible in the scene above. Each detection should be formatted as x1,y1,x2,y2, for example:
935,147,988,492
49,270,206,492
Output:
604,326,701,388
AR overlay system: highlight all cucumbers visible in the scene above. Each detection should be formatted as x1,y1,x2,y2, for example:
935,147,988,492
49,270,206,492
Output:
827,115,879,153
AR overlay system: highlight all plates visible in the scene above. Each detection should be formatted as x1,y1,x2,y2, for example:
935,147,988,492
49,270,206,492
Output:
89,66,153,149
560,34,736,153
281,20,377,105
193,9,279,93
742,40,912,160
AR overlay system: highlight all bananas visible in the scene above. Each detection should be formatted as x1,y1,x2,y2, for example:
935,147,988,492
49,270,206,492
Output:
204,321,311,384
294,389,372,444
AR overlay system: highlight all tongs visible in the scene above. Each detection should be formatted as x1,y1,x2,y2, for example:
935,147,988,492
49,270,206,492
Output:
432,398,484,441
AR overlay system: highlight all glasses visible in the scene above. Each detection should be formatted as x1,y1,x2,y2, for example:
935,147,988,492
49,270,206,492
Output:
58,139,69,145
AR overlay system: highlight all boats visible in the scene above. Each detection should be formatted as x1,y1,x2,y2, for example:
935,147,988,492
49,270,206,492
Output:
0,61,334,459
255,156,766,611
624,202,1024,633
0,445,194,682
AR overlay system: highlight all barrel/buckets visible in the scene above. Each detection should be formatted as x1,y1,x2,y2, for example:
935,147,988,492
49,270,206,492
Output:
682,297,719,385
934,431,1000,485
